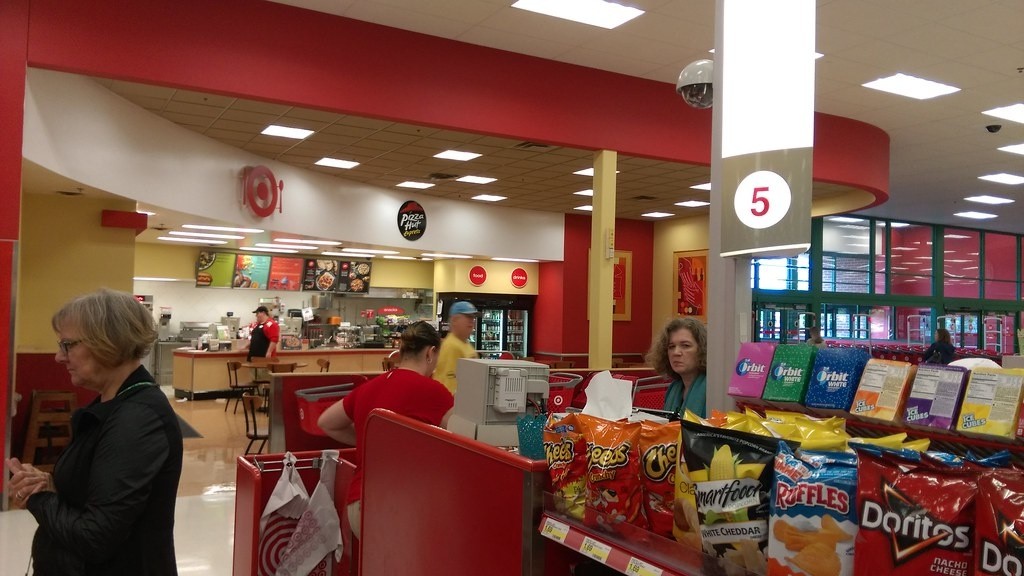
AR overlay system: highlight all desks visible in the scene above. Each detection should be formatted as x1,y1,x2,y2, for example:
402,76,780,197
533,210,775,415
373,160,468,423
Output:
269,371,388,453
243,361,309,397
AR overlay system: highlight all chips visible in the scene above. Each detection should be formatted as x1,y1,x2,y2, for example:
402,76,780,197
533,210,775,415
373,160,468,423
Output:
772,513,854,576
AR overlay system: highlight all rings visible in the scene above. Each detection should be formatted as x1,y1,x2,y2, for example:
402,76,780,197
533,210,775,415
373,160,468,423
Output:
15,490,25,500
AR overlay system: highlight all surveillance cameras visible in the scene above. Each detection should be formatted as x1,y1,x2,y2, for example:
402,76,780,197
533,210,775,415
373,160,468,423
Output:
986,125,1002,132
676,59,716,109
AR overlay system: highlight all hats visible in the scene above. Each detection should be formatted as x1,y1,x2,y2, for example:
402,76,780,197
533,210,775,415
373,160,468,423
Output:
252,306,268,313
449,302,481,317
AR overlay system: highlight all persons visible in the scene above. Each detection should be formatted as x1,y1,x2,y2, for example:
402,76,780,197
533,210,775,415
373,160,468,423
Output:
318,320,455,446
644,317,707,418
5,289,183,576
246,306,280,411
924,329,955,364
806,327,827,347
431,301,478,394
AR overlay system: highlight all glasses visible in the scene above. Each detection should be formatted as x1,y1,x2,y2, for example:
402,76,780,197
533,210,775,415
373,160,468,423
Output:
56,337,86,356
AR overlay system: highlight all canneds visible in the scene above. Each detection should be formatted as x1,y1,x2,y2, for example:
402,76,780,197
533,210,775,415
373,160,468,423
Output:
482,323,524,342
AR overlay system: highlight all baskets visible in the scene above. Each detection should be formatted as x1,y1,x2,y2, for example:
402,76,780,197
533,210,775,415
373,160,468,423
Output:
516,414,549,460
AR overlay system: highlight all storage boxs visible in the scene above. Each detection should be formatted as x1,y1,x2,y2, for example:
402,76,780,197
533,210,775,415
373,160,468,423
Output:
761,344,819,408
849,358,915,430
903,361,967,437
956,367,1024,445
804,346,869,414
726,340,778,409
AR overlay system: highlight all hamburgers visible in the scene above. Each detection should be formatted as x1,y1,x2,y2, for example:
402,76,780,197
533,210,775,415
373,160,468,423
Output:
196,272,212,286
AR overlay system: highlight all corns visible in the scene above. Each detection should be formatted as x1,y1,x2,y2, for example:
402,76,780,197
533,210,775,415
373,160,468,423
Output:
704,445,739,482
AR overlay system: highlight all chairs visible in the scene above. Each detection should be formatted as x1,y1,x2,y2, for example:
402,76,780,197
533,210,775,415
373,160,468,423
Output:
241,391,268,457
224,361,253,414
316,359,331,373
265,364,299,414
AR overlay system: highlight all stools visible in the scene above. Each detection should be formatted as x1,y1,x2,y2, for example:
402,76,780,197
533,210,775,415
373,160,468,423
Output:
21,390,76,474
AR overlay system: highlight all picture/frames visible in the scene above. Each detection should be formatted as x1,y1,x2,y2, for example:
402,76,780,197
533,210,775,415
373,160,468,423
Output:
587,248,632,321
670,249,707,325
